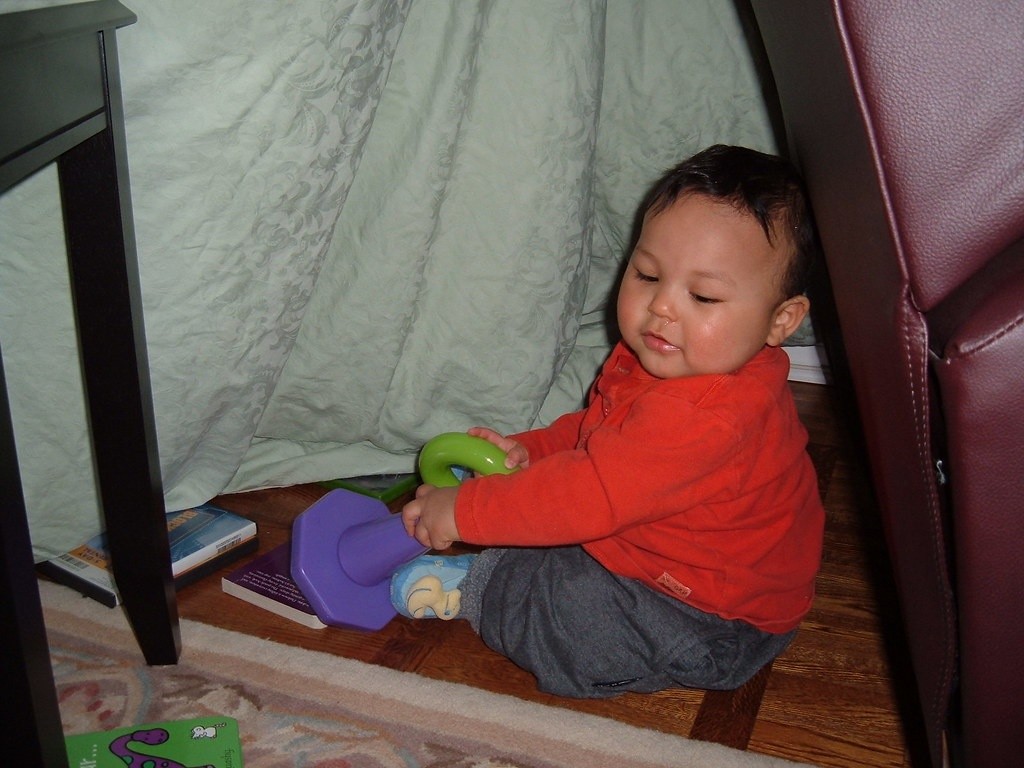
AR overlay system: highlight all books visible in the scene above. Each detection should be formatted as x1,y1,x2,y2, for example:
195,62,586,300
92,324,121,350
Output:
35,502,328,631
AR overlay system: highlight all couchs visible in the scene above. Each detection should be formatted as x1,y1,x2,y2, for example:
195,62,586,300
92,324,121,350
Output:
750,0,1024,768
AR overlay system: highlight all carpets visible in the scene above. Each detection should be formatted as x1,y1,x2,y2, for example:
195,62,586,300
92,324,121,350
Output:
37,572,819,768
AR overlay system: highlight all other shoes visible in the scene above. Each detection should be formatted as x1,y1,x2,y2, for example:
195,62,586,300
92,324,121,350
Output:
389,553,480,620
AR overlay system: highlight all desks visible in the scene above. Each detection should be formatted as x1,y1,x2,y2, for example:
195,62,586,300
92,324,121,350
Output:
0,0,181,768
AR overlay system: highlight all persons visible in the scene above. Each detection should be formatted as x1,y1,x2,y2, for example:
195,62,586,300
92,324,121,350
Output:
390,146,824,699
